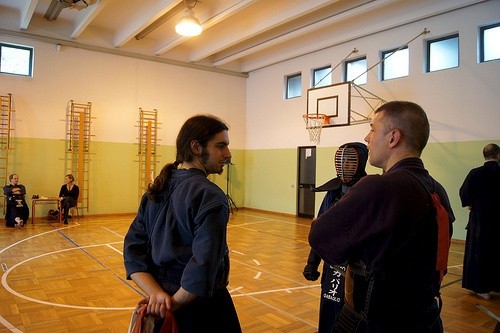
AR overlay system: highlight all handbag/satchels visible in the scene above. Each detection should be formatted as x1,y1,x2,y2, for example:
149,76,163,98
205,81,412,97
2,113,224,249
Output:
48,209,60,219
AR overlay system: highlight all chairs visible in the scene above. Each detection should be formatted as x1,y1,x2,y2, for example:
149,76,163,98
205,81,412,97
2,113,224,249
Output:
69,203,80,224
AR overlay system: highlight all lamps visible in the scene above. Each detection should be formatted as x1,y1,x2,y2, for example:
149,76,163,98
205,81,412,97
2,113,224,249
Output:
60,0,97,10
175,0,202,37
44,0,63,21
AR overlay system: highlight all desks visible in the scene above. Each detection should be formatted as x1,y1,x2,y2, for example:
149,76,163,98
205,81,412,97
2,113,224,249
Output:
30,198,62,226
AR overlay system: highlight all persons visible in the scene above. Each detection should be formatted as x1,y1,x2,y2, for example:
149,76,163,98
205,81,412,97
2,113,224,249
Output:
2,174,29,229
123,115,242,333
50,174,79,225
302,142,369,333
308,100,455,333
459,143,500,299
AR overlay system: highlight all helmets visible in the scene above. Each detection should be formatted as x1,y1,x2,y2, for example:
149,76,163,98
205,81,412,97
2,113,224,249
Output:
333,142,368,185
